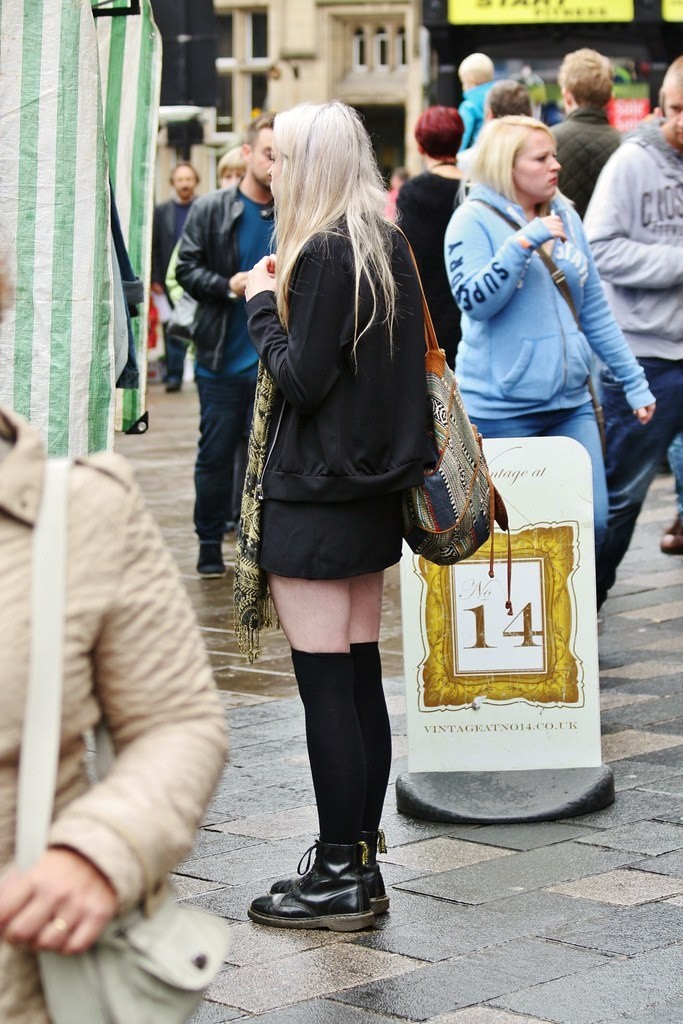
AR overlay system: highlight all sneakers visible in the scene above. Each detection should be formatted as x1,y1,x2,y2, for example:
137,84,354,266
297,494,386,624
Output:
196,544,228,579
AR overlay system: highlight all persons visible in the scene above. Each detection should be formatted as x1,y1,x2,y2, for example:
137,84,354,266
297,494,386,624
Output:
453,79,535,187
391,104,471,374
161,146,254,380
148,161,202,391
445,114,658,563
457,53,497,153
547,46,625,223
0,250,227,1021
581,53,683,618
243,100,440,931
174,112,279,580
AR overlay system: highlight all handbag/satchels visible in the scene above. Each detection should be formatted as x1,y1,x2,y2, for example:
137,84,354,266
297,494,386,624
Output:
35,906,225,1024
383,216,505,567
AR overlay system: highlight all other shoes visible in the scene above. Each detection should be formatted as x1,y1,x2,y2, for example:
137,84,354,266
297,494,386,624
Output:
661,520,683,554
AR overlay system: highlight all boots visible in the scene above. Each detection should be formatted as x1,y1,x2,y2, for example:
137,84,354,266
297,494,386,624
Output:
267,829,390,915
247,839,376,932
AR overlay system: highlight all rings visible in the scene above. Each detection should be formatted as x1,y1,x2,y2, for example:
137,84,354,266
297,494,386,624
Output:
50,913,69,937
